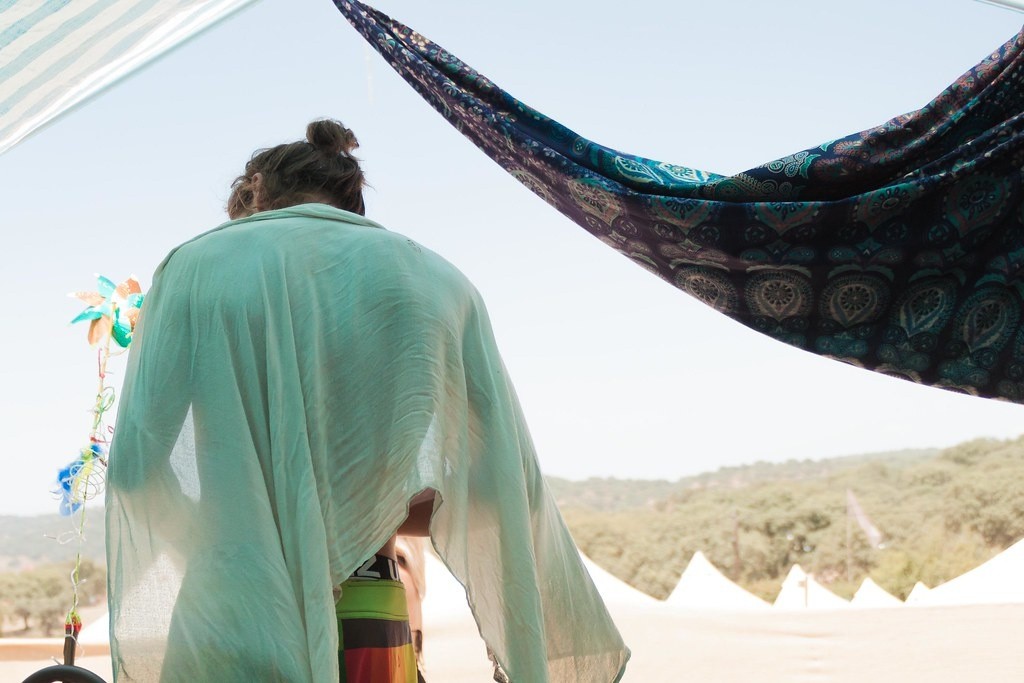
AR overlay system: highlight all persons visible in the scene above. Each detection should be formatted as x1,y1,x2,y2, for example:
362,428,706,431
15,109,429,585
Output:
107,120,544,683
